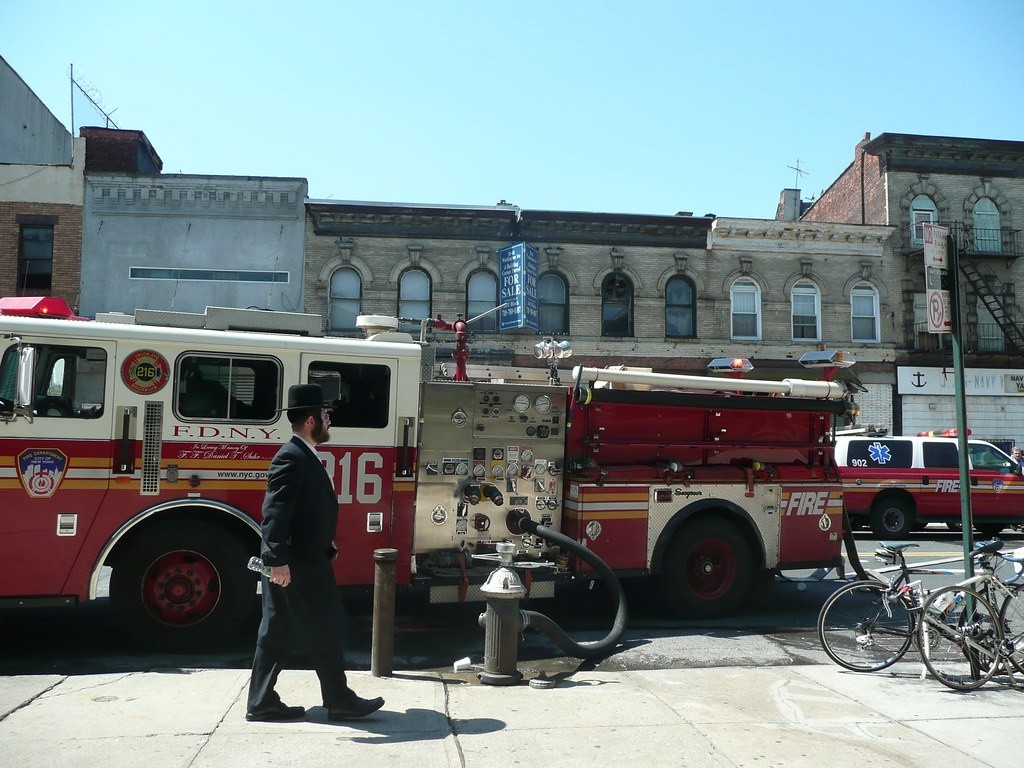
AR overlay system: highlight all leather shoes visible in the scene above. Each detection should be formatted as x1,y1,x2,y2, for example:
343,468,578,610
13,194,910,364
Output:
246,692,305,721
328,691,384,721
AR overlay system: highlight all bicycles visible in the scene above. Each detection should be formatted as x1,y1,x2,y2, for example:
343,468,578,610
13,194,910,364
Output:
818,537,1024,674
916,540,1024,691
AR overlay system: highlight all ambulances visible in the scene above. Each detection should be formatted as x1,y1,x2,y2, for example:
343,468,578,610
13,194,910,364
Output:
830,422,1024,539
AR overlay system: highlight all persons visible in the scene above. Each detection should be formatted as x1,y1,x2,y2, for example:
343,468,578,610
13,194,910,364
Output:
1005,445,1024,475
245,385,385,722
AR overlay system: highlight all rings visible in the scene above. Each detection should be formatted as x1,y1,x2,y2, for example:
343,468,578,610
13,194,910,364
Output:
272,577,276,581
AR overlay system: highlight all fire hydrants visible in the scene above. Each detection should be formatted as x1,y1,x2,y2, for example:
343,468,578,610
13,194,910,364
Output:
471,542,556,681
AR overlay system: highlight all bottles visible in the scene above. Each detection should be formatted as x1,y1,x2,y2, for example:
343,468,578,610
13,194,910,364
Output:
934,592,959,612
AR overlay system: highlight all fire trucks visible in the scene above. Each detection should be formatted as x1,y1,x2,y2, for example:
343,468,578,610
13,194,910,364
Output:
0,295,867,655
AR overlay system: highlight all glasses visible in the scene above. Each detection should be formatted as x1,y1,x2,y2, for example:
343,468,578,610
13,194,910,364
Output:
319,413,329,421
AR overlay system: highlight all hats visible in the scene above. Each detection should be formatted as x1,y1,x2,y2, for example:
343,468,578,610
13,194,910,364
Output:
274,384,338,411
